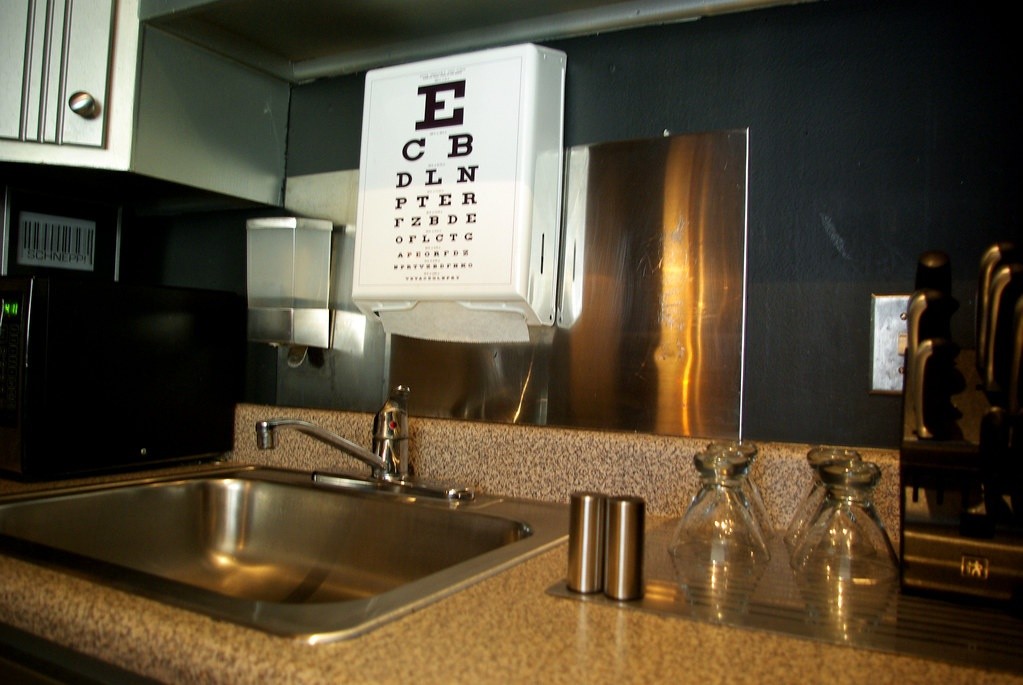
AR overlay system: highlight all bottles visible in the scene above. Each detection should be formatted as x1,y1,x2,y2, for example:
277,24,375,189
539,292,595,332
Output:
783,444,898,642
667,437,786,627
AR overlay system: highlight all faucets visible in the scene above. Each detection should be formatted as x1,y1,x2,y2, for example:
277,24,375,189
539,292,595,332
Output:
254,382,415,486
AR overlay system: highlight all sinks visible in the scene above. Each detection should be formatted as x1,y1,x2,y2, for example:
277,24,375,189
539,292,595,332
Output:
0,465,571,648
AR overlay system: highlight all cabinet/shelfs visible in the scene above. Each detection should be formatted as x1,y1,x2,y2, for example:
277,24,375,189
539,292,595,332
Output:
0,1,289,208
